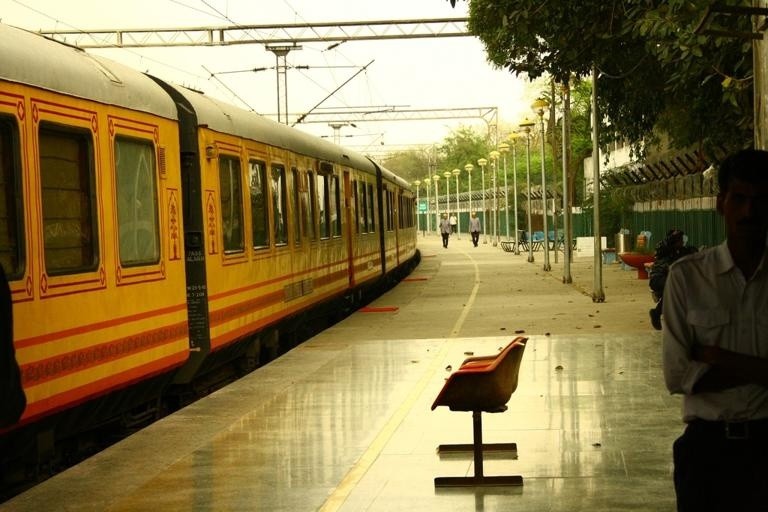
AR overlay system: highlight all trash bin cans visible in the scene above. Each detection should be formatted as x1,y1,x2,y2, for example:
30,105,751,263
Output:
615,233,632,262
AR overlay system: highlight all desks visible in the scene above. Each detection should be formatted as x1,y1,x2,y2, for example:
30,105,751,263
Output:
620,254,656,279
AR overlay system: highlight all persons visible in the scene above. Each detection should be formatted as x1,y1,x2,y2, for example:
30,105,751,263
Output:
448,212,458,236
439,213,451,249
648,247,699,332
468,211,481,248
648,230,684,299
660,150,767,510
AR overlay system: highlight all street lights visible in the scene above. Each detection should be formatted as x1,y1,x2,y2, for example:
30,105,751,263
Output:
415,96,552,274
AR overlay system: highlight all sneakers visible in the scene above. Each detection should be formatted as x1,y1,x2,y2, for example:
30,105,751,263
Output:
650,307,663,331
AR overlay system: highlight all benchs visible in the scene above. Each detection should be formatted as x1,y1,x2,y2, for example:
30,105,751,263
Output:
601,248,616,266
501,230,576,252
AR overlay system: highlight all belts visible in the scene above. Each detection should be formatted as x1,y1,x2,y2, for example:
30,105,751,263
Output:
689,417,768,439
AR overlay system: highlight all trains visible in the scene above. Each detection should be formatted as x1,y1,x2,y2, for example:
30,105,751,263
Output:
1,12,416,488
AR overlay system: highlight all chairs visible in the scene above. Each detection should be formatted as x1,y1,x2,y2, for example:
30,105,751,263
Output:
432,336,530,486
